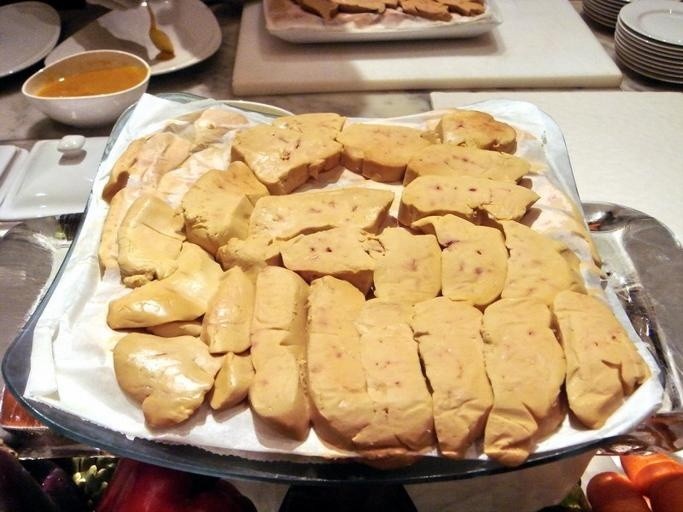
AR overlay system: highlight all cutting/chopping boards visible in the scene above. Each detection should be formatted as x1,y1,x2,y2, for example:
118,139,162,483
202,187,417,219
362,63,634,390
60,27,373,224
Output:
231,0,629,100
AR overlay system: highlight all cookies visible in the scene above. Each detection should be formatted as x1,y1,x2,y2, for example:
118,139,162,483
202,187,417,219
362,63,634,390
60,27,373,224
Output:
290,0,486,22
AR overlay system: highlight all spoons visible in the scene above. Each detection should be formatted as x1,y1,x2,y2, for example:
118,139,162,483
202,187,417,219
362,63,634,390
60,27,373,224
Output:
144,1,174,58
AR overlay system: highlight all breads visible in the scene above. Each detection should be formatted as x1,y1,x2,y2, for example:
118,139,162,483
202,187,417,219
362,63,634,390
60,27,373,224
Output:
98,107,683,512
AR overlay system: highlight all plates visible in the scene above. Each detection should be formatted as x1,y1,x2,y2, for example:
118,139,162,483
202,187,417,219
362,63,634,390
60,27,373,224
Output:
0,90,666,490
43,0,222,79
0,0,62,82
582,0,682,87
261,0,502,46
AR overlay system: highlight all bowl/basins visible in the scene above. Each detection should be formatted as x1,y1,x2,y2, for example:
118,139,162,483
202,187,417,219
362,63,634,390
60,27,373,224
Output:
21,49,151,129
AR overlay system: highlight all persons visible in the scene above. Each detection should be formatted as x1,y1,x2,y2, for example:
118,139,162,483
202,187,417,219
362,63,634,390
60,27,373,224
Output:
87,0,143,13
